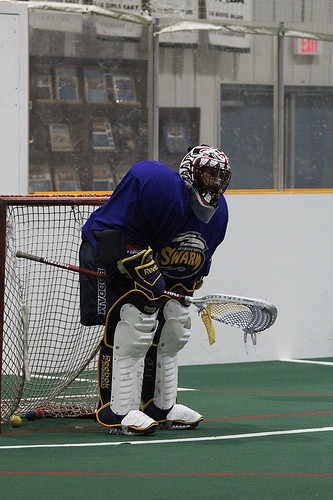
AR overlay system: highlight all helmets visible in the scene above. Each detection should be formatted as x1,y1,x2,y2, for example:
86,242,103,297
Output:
178,144,232,224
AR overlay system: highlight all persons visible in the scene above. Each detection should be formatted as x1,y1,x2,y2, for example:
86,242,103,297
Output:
79,145,231,436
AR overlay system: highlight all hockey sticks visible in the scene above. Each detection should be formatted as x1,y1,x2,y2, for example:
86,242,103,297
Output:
16,250,279,358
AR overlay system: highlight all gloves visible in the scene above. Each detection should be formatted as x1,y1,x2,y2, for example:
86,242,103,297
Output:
119,249,164,314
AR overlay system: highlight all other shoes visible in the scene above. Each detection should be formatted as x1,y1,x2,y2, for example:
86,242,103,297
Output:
151,404,205,431
106,411,159,435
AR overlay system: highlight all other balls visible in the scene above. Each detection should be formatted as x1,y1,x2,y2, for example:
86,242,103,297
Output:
11,416,23,428
25,410,36,421
35,408,44,418
